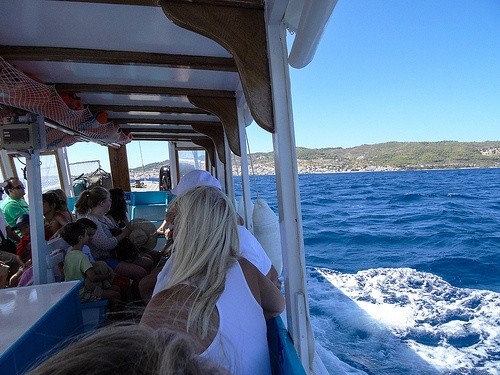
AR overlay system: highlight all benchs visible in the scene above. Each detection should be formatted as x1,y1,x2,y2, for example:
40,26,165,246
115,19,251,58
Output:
267,315,307,375
81,299,109,331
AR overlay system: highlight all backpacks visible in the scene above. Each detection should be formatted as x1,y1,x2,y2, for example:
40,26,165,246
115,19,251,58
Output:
110,228,137,261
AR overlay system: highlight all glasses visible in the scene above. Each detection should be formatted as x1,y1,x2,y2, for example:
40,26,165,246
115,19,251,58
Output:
13,184,23,190
101,199,112,203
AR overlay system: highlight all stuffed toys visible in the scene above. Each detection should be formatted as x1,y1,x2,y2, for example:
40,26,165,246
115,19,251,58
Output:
81,260,116,301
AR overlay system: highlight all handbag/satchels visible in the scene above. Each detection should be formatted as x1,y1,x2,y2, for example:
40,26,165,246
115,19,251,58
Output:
72,173,87,196
88,168,112,192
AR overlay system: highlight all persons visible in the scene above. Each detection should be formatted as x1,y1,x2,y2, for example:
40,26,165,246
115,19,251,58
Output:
139,170,282,306
160,166,171,191
18,320,232,375
157,207,175,234
1,176,155,318
137,186,285,375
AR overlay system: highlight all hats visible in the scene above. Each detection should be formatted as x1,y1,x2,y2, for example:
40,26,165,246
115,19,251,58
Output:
0,182,7,188
170,170,221,196
85,261,114,290
125,218,157,251
11,214,29,231
